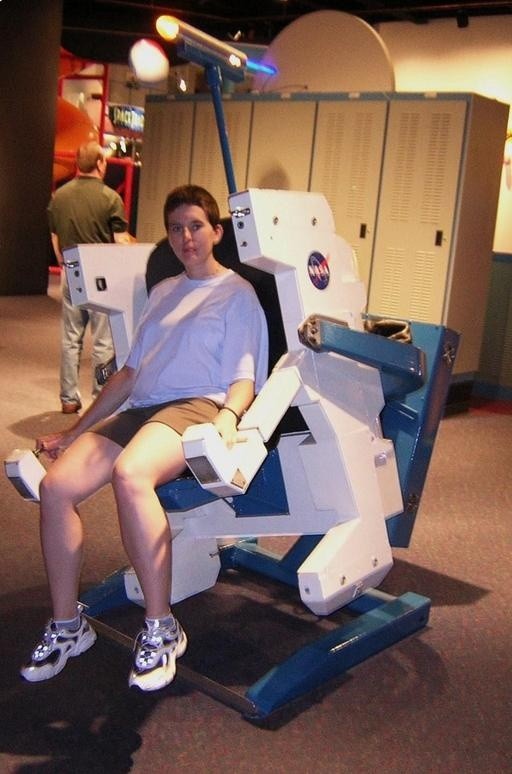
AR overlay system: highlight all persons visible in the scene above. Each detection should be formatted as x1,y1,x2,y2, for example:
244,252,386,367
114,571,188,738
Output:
46,139,140,415
16,182,274,699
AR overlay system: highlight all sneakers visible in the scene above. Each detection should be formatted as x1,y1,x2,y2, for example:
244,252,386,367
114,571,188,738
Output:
128,618,187,696
19,615,97,682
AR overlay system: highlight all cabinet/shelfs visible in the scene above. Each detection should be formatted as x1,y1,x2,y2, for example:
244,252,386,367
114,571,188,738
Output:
308,101,388,313
245,101,316,191
137,98,195,244
190,99,253,224
367,98,510,374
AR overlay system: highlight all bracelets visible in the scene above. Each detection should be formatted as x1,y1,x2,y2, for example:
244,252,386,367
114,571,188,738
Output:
218,406,241,424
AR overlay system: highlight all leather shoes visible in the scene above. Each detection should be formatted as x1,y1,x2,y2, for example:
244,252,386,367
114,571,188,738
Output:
63,401,81,413
